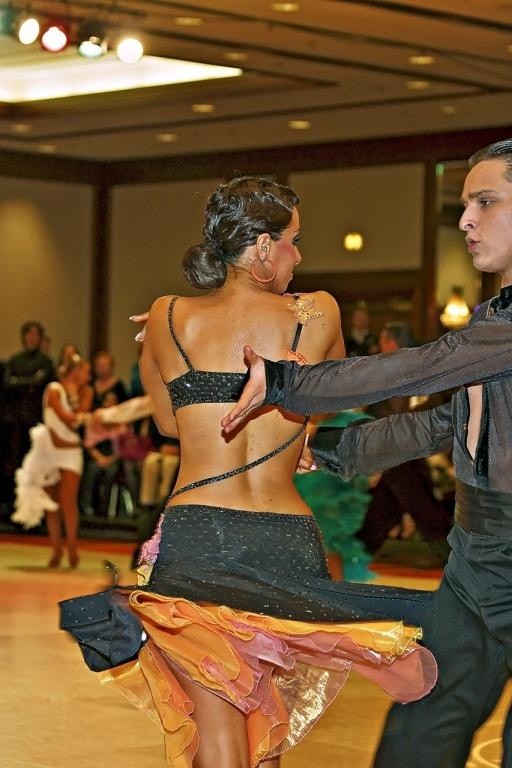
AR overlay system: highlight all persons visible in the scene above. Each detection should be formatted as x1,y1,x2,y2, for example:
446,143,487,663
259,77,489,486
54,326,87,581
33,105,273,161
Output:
10,353,92,567
220,141,512,768
57,177,437,768
344,301,467,558
1,322,179,543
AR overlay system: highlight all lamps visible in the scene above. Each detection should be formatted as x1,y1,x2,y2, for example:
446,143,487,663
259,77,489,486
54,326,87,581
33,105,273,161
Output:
0,0,147,64
